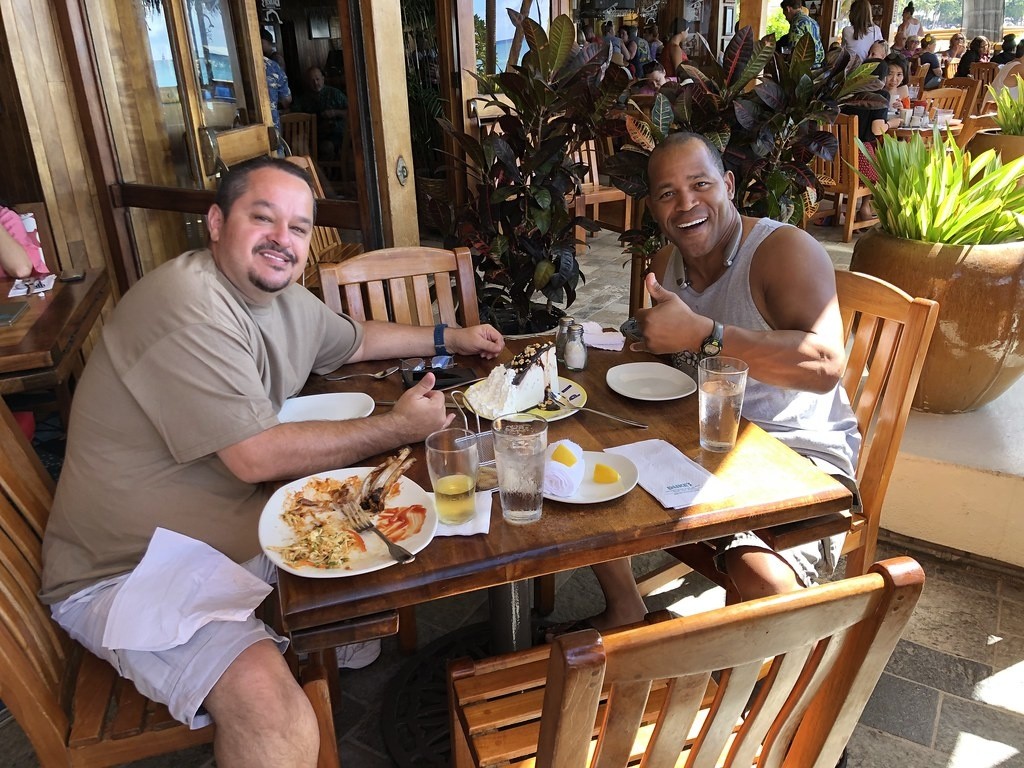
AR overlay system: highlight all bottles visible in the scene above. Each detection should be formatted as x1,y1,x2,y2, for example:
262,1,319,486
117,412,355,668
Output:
555,317,574,363
564,324,588,372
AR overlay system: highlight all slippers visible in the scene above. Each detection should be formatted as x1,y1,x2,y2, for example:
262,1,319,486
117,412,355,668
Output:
540,617,598,646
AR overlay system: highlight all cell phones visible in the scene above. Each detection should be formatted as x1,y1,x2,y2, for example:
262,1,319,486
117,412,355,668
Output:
60,270,86,282
401,366,478,388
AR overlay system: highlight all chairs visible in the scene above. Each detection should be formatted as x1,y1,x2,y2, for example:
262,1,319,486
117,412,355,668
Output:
319,243,481,335
277,108,358,187
0,399,340,768
442,556,924,768
660,266,940,605
476,51,1000,256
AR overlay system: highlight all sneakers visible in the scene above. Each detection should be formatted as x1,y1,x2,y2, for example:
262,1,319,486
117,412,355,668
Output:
298,639,382,671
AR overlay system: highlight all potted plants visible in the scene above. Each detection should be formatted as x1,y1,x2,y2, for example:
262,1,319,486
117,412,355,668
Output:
960,73,1024,194
848,121,1024,413
429,0,633,356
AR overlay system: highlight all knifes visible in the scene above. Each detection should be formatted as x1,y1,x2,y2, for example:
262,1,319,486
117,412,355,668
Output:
374,400,464,408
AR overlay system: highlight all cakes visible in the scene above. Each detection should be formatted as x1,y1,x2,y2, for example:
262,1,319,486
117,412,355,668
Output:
467,340,558,418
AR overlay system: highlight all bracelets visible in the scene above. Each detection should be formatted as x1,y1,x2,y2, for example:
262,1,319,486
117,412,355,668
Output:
434,324,452,355
887,122,891,129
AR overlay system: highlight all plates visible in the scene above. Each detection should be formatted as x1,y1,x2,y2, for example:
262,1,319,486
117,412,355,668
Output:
605,362,697,400
950,119,962,125
276,392,374,423
258,467,438,578
542,451,639,503
463,375,588,423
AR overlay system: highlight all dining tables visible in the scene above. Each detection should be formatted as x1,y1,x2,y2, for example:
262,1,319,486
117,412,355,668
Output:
885,123,964,141
260,325,852,660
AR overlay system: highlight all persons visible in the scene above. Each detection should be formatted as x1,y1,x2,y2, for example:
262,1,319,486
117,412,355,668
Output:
260,28,292,158
299,66,349,182
544,130,862,768
954,35,991,78
582,26,604,61
601,20,630,65
821,0,926,233
987,39,1024,101
781,0,825,137
629,25,664,75
689,35,697,56
0,205,51,278
659,17,689,76
990,33,1016,81
618,25,644,78
915,33,945,88
941,32,966,78
411,33,438,63
38,158,506,768
578,29,597,81
603,62,681,122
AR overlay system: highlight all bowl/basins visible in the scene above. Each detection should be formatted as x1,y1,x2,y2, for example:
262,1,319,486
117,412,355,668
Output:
887,113,900,120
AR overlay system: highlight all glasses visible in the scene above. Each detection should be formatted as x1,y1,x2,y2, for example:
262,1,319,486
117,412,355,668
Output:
875,39,886,44
910,40,919,46
955,37,964,41
887,52,907,60
398,353,457,372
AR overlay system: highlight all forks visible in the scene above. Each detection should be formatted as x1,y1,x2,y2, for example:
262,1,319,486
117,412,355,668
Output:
343,500,415,565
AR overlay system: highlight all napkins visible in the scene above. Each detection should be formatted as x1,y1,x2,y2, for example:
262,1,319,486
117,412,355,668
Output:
424,488,494,539
104,527,275,652
578,320,625,351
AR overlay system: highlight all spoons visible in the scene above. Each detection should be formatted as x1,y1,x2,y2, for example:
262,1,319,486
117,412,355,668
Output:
549,391,649,428
324,367,399,380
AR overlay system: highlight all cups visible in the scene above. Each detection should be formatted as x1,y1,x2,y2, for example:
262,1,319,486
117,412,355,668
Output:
938,109,953,130
912,116,923,128
914,107,925,117
425,428,479,525
889,95,899,112
899,109,913,127
936,54,942,63
492,412,548,525
909,87,920,98
698,356,750,453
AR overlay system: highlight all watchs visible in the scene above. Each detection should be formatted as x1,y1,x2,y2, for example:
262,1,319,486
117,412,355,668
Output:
700,322,724,357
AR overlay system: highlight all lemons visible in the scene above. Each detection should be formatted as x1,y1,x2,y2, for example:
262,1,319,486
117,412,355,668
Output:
551,444,578,467
594,463,622,483
